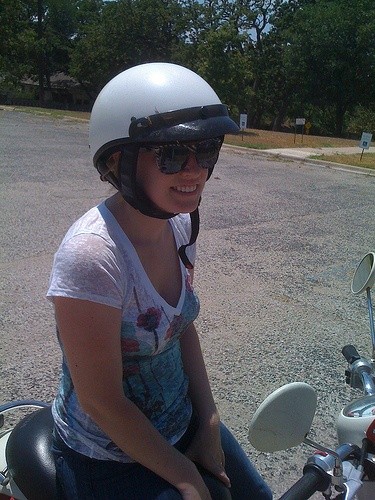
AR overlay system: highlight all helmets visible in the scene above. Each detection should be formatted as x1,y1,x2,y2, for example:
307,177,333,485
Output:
89,62,240,167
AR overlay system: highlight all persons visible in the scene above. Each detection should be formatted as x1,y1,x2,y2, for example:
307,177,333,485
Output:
45,63,275,500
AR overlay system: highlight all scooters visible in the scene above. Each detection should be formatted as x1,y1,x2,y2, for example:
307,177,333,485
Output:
0,250,375,500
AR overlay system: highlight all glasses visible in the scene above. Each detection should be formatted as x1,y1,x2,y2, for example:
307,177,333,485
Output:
135,137,221,176
